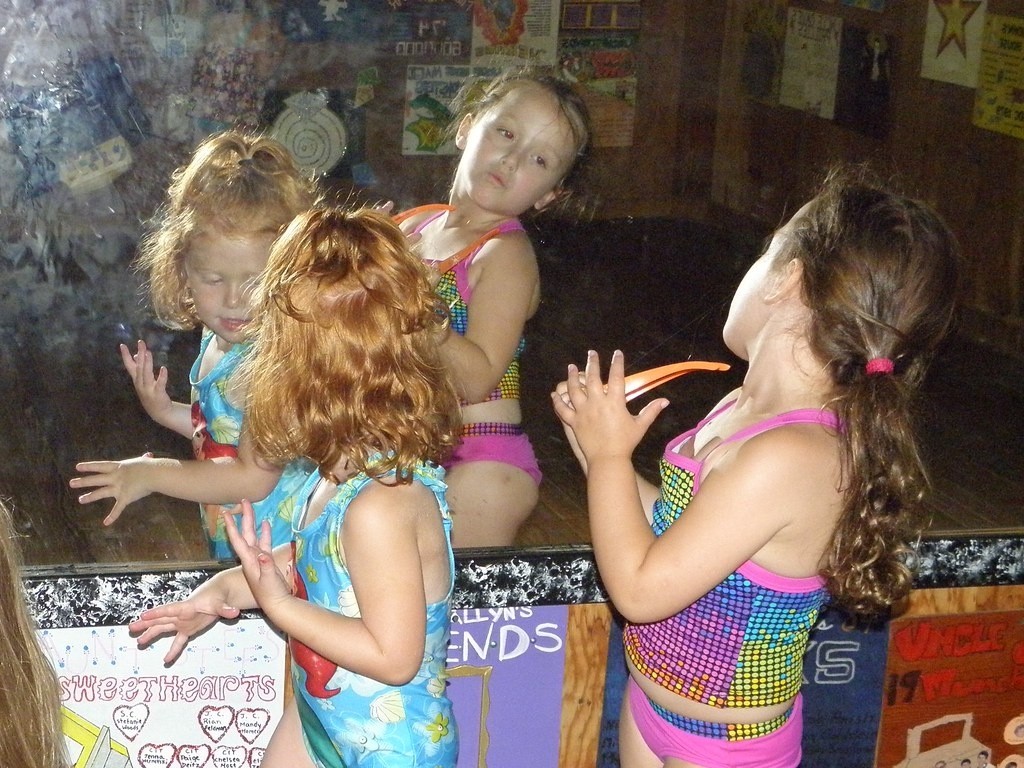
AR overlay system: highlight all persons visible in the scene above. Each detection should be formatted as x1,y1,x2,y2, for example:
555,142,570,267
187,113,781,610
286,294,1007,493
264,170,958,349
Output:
70,130,319,563
545,163,975,768
129,206,468,768
852,35,893,133
385,65,588,548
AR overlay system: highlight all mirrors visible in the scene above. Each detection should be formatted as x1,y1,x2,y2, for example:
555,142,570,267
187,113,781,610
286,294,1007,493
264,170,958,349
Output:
0,0,1024,574
